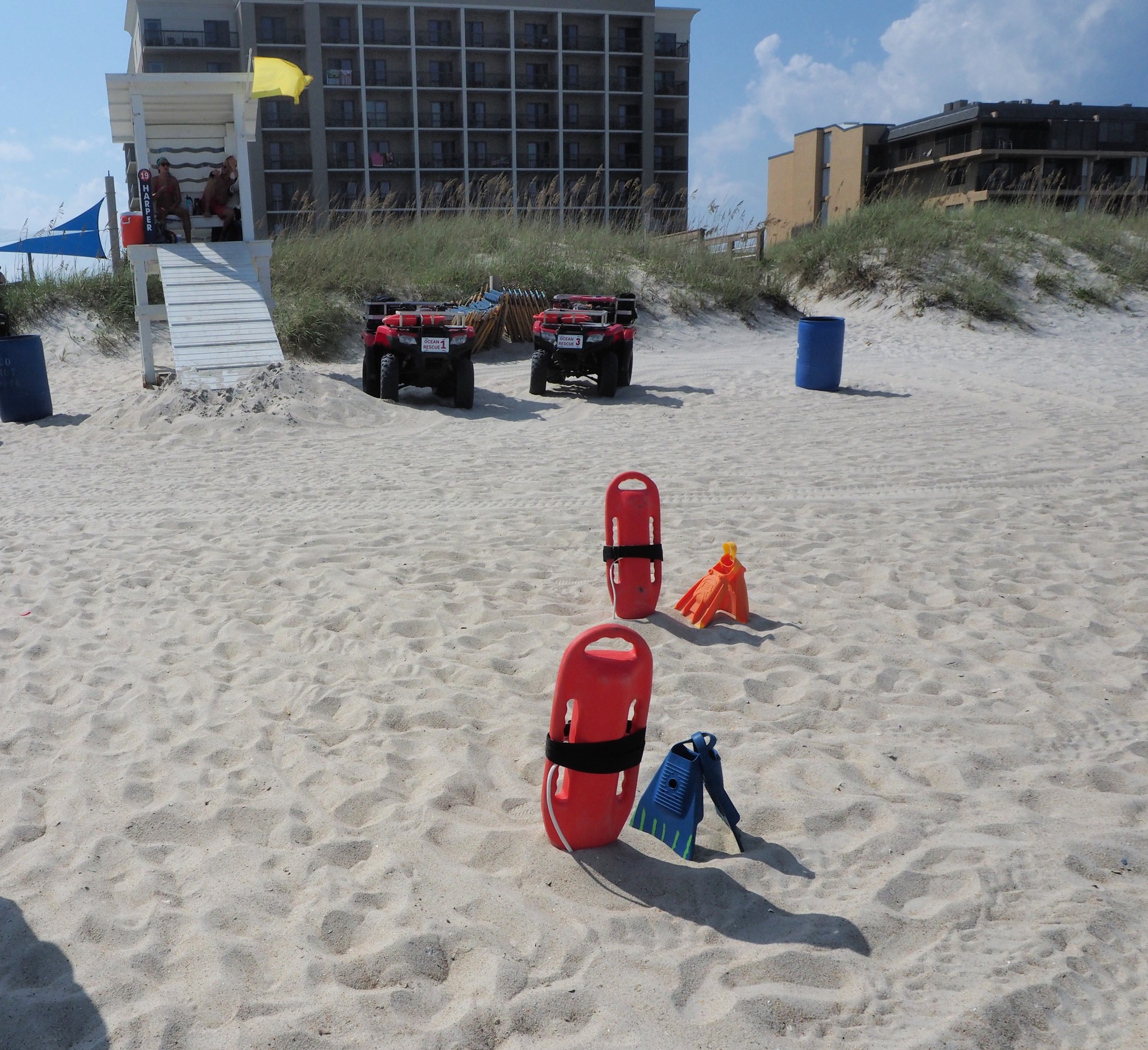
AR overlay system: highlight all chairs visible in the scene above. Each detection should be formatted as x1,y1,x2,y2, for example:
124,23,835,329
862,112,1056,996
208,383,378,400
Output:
998,137,1012,149
182,38,198,46
167,37,174,46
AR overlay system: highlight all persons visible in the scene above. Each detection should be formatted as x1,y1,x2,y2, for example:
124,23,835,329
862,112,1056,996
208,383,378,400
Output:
152,158,191,243
200,154,238,230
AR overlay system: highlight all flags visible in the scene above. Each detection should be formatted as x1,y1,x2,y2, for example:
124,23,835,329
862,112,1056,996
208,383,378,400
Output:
251,56,312,105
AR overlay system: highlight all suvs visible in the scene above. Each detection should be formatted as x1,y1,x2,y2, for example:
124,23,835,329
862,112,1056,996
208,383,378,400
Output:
360,296,476,409
528,292,637,396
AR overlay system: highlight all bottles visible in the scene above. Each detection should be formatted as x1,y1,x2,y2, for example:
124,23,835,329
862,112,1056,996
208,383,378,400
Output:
194,198,203,215
185,195,194,216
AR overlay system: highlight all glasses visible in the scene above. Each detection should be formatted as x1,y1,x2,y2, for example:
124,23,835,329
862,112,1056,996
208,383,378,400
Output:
221,164,228,169
159,162,170,167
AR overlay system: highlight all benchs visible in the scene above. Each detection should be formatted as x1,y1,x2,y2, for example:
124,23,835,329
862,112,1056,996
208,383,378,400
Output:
166,215,224,229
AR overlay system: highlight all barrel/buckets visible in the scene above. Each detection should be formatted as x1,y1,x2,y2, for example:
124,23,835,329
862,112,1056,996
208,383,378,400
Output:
119,211,144,248
0,334,53,424
795,316,846,392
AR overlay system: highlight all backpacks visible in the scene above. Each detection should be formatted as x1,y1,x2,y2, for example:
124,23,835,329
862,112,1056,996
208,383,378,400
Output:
155,219,178,244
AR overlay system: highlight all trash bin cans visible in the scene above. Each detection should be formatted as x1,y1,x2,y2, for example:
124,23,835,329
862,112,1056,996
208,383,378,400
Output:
0,335,53,423
795,316,845,390
119,211,145,248
